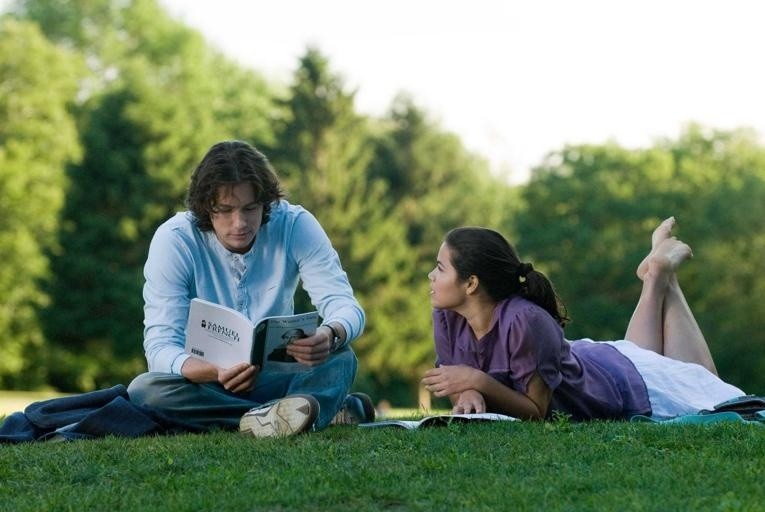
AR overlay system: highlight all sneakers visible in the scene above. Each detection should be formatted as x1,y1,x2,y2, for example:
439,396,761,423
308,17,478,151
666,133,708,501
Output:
239,393,320,440
331,392,375,426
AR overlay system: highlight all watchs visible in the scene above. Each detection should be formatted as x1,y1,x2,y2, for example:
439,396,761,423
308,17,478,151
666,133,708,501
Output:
321,322,343,355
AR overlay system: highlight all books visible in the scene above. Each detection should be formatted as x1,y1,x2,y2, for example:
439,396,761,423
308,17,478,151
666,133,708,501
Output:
358,411,524,430
183,297,319,373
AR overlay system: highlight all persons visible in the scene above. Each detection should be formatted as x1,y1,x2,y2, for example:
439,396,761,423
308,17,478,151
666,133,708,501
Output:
420,215,749,424
126,141,374,438
267,327,310,363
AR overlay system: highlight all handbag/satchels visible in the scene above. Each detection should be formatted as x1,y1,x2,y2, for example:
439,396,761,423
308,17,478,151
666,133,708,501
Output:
698,395,765,424
